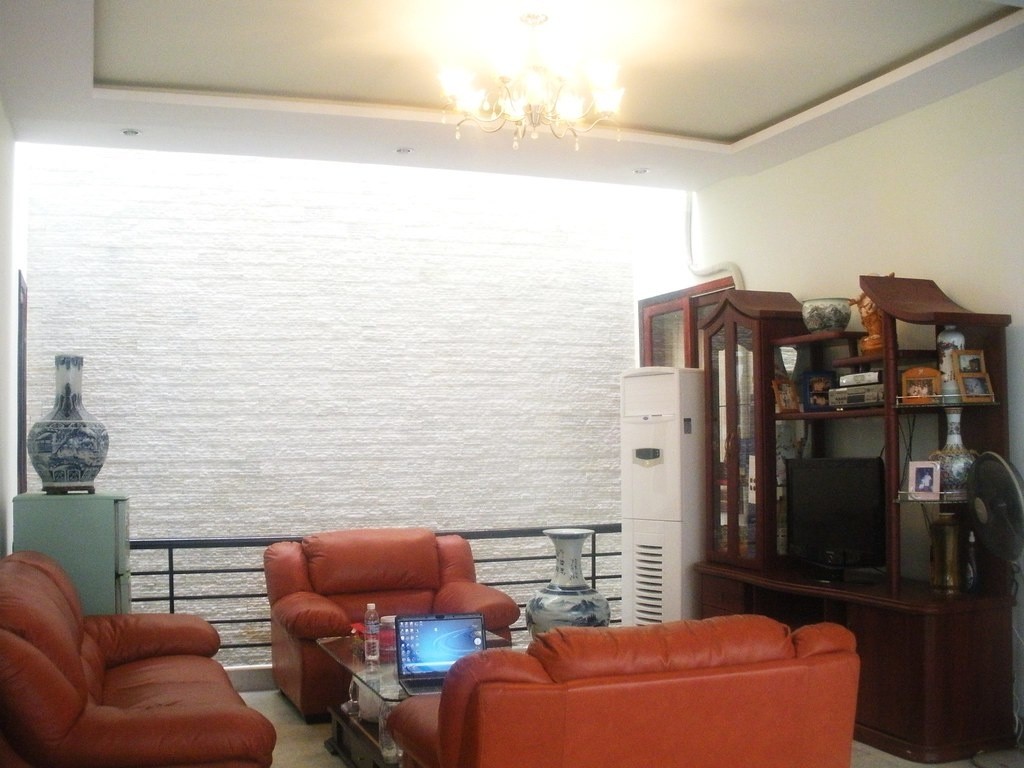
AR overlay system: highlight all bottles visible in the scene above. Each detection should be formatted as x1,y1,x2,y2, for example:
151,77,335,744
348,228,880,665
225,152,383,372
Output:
364,603,381,661
525,527,612,641
377,693,398,763
938,325,966,403
26,352,110,494
929,408,976,505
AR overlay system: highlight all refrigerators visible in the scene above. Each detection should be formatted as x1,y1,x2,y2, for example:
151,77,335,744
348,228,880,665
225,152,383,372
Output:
12,494,134,616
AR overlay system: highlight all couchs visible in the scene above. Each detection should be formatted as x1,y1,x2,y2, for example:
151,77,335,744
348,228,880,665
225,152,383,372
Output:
387,614,862,767
264,528,521,724
1,550,277,768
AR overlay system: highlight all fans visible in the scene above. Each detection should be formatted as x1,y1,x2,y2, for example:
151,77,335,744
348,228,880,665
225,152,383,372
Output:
966,451,1024,768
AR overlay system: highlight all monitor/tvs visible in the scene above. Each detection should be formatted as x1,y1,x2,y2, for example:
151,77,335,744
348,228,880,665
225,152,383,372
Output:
783,458,886,585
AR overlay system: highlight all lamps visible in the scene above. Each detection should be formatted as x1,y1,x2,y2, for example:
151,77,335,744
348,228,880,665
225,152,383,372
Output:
435,6,630,152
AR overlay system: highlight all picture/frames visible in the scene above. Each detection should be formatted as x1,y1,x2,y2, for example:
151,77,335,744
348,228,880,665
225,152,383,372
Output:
910,459,941,502
798,371,839,413
952,349,996,403
901,366,942,403
769,378,801,413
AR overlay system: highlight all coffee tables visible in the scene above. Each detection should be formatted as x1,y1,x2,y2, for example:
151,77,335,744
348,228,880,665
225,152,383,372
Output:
317,626,532,768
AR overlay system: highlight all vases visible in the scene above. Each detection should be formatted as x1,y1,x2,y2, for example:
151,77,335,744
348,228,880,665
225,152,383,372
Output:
937,324,966,404
929,511,969,599
25,354,109,495
929,408,972,501
800,297,852,333
524,528,610,638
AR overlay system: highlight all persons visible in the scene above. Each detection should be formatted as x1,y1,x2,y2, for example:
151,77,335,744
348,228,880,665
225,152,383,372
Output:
782,389,792,408
909,380,929,396
969,378,983,397
919,471,931,492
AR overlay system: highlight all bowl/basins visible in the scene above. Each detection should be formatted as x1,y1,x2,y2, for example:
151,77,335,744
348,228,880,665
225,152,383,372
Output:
800,297,851,334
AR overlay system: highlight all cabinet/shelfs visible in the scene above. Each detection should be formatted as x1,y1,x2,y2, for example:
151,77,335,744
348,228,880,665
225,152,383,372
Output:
696,273,1015,764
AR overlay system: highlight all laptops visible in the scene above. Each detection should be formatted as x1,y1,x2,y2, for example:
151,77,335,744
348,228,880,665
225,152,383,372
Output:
394,611,487,694
